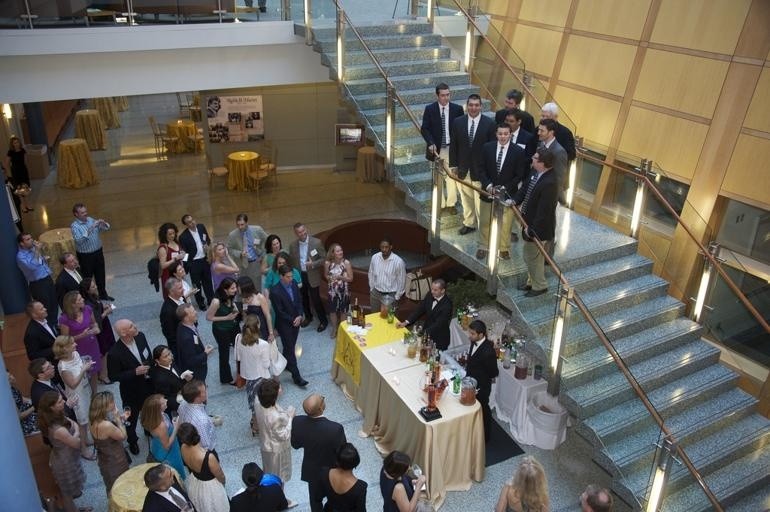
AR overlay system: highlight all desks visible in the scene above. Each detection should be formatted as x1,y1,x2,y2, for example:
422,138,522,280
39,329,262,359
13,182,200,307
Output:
55,96,129,189
332,311,486,511
38,228,80,285
450,306,570,450
356,147,385,183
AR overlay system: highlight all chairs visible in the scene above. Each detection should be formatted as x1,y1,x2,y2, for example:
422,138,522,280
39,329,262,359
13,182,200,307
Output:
149,91,204,158
0,0,261,29
205,140,279,199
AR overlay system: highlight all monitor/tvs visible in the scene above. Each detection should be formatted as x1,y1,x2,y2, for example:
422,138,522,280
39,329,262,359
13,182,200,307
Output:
335,124,365,145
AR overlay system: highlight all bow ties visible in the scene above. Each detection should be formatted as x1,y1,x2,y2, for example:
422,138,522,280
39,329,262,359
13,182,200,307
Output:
433,299,438,302
474,343,477,346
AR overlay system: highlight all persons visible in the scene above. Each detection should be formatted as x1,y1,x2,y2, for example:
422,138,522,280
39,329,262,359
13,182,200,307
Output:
7,233,230,511
72,203,115,302
245,0,266,13
395,279,499,442
231,313,426,512
421,83,575,297
7,137,35,215
492,455,613,511
207,97,221,119
155,214,405,388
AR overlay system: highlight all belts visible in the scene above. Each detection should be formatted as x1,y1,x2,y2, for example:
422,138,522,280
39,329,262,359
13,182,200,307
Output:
375,288,395,296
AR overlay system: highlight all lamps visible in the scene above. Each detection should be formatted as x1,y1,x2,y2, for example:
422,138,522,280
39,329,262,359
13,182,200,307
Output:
385,108,391,166
337,34,342,80
692,255,714,322
565,149,579,208
519,88,525,111
464,21,471,72
304,0,308,24
646,436,676,511
486,202,505,299
430,157,446,258
427,0,432,22
630,175,648,238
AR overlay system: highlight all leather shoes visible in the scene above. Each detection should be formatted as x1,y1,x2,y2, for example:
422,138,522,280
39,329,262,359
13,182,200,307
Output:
518,284,532,291
99,296,114,301
287,500,298,508
300,318,337,339
476,249,487,260
457,226,475,236
198,305,207,311
129,442,139,455
525,289,549,298
510,233,520,242
500,251,510,260
294,376,308,387
447,207,457,216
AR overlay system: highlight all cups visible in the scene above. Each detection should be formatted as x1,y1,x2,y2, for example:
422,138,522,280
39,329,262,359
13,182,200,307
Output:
33,239,48,250
171,410,178,422
411,464,422,478
124,406,131,416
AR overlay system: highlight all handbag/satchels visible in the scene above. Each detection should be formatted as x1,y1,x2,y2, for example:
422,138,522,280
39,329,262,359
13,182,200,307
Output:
150,244,168,279
146,453,158,464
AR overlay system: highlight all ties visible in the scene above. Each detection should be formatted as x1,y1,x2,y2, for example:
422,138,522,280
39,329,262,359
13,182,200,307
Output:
441,108,446,149
521,174,538,216
242,231,249,269
73,271,82,282
469,120,475,148
169,490,190,511
511,135,514,143
496,146,504,177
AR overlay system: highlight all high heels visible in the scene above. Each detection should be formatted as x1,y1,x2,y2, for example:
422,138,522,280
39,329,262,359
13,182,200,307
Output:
250,418,254,428
97,375,113,384
236,375,245,388
252,428,259,437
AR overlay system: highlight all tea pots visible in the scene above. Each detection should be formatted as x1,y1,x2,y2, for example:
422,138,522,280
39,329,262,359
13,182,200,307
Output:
460,375,478,405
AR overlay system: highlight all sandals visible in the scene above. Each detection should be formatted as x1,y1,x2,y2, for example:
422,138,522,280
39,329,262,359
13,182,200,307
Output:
79,506,93,512
221,379,236,386
80,452,97,461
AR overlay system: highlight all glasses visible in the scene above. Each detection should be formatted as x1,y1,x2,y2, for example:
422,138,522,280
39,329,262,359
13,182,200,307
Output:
319,396,325,410
41,362,52,373
532,155,538,160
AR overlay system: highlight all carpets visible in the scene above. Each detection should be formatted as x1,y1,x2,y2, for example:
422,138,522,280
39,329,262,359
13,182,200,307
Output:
485,418,526,467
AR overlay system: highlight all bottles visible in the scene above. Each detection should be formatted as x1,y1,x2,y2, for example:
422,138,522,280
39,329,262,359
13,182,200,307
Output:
348,297,366,328
534,365,544,382
527,361,533,375
405,324,447,383
456,301,479,331
387,304,395,324
428,379,436,414
495,335,527,379
453,370,461,393
